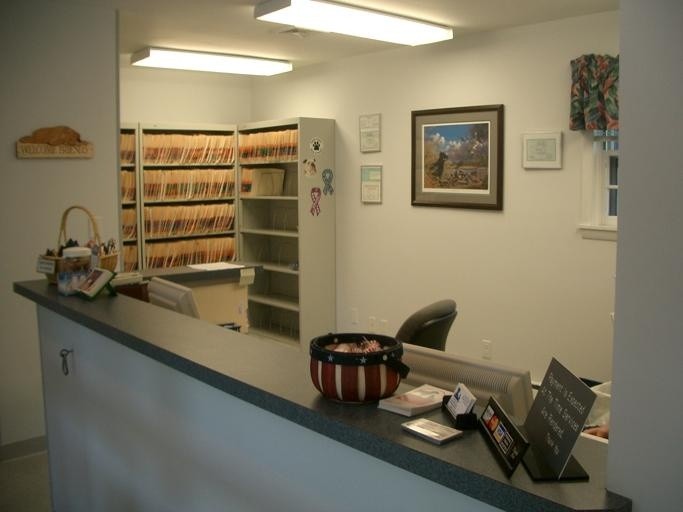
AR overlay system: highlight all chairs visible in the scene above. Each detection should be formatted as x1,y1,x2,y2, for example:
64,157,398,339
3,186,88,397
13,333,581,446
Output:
394,295,458,369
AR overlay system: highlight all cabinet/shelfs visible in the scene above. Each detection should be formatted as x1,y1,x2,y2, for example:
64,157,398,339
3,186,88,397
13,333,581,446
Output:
119,125,142,276
238,117,335,346
140,121,238,270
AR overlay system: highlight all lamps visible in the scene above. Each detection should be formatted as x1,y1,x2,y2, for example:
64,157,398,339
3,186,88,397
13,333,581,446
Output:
127,46,296,78
252,1,456,46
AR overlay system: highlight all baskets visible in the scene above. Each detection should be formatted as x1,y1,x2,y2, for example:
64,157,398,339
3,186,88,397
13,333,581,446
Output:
42,252,119,288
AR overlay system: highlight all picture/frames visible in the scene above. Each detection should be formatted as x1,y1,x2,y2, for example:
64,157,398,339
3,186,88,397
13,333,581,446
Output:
411,105,509,210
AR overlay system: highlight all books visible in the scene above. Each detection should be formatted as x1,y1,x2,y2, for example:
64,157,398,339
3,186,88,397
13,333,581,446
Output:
238,127,297,163
119,132,235,269
401,418,463,446
241,167,286,197
378,383,453,417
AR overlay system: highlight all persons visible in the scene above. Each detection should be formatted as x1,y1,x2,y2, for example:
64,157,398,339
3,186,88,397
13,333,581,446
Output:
584,422,610,440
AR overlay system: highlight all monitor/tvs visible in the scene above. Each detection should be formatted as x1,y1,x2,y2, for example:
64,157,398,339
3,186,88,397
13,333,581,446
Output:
399,343,534,429
147,276,200,320
